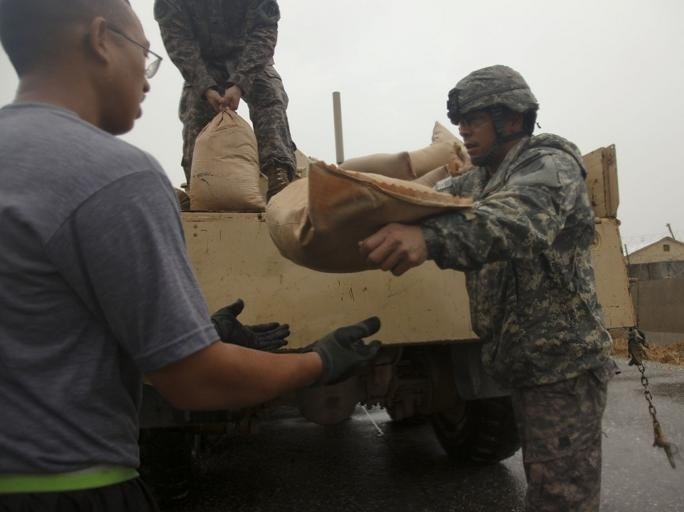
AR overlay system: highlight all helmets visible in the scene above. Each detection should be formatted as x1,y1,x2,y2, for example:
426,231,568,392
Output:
448,66,539,126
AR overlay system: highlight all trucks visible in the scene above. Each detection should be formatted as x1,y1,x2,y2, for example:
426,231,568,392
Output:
139,141,639,467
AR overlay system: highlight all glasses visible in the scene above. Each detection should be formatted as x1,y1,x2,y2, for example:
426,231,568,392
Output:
104,20,162,78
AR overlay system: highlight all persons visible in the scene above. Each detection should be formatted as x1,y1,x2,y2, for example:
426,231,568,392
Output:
0,1,382,512
154,0,297,211
359,65,613,512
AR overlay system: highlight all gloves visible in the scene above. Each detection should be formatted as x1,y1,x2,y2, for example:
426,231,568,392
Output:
304,316,384,388
211,299,291,351
